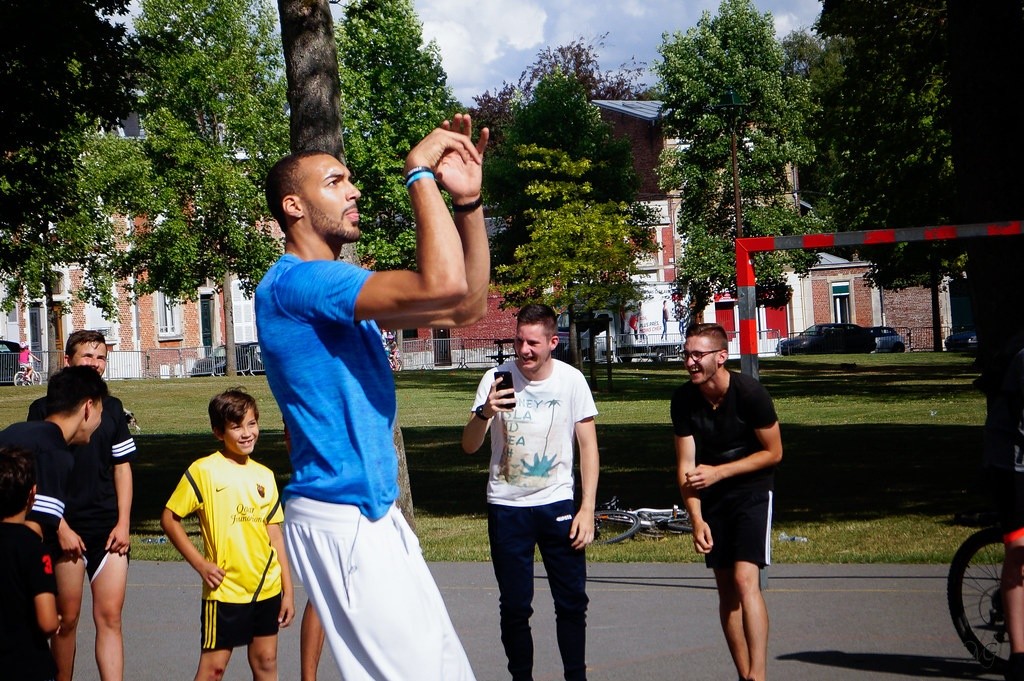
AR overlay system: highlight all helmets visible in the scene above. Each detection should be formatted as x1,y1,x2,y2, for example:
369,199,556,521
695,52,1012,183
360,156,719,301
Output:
20,341,28,348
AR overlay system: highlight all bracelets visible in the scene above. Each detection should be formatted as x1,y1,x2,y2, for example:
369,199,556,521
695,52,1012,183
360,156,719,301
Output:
404,166,435,190
451,193,483,213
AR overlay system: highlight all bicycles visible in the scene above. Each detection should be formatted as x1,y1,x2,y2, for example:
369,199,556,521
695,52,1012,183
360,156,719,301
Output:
591,494,693,546
14,360,42,387
381,350,406,375
946,526,1011,675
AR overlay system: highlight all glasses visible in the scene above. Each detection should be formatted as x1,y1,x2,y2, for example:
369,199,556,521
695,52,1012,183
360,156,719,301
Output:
680,350,722,362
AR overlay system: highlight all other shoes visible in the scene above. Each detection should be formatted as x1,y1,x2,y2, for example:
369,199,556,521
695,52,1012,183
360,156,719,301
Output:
24,375,30,381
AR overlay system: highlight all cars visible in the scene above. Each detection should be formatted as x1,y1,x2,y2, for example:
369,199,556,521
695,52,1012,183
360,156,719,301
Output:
0,340,22,383
944,330,977,352
776,323,877,356
861,327,905,353
190,341,266,378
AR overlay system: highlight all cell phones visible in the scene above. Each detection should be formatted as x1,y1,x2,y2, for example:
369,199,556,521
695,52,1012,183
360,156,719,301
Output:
493,371,516,409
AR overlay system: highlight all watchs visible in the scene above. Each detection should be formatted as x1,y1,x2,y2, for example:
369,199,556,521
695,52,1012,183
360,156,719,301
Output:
476,404,489,421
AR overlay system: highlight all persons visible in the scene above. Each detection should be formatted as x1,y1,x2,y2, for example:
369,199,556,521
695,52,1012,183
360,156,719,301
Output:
160,385,296,681
983,347,1024,681
661,299,690,341
670,322,783,681
629,301,646,344
462,301,600,681
381,329,401,371
300,597,325,681
0,328,136,681
253,112,491,681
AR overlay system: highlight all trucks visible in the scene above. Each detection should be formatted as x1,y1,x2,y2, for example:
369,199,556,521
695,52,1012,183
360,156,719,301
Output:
551,282,691,365
